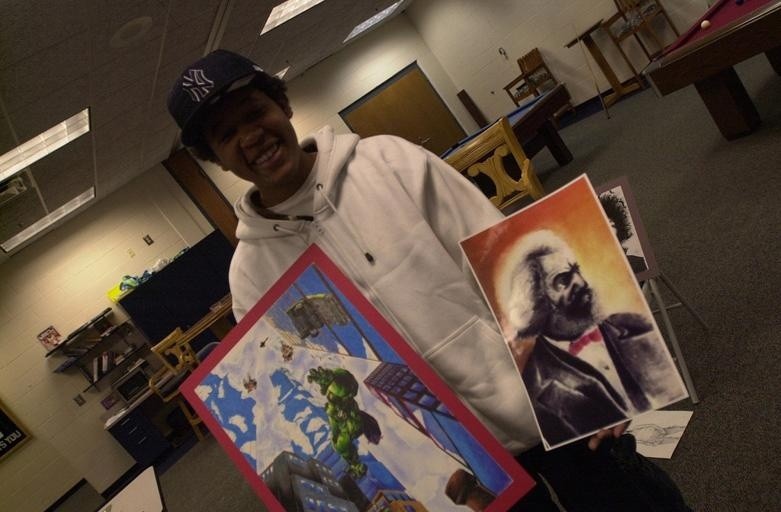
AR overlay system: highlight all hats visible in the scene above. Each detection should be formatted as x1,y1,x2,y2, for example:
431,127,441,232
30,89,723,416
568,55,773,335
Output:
167,48,270,147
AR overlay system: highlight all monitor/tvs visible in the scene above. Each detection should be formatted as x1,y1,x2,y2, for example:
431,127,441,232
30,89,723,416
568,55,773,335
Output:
118,229,235,368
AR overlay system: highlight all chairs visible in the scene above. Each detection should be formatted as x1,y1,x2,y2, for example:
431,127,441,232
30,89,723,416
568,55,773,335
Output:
146,326,207,441
440,116,548,221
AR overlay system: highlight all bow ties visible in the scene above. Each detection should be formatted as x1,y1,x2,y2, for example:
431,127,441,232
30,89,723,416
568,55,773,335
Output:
569,328,602,355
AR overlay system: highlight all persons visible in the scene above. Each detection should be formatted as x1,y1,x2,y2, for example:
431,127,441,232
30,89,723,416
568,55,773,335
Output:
598,196,648,289
167,45,687,512
493,228,683,443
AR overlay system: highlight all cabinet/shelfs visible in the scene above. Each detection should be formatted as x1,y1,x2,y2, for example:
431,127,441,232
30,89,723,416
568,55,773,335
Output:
44,306,147,393
504,64,578,129
603,1,680,90
105,373,209,475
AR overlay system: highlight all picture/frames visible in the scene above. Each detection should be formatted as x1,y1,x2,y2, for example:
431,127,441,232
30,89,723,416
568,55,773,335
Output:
0,398,31,463
178,240,539,512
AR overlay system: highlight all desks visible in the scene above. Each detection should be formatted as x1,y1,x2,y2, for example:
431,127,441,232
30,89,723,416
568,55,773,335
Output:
175,293,236,350
92,461,167,512
439,82,576,195
563,18,642,108
642,0,781,142
